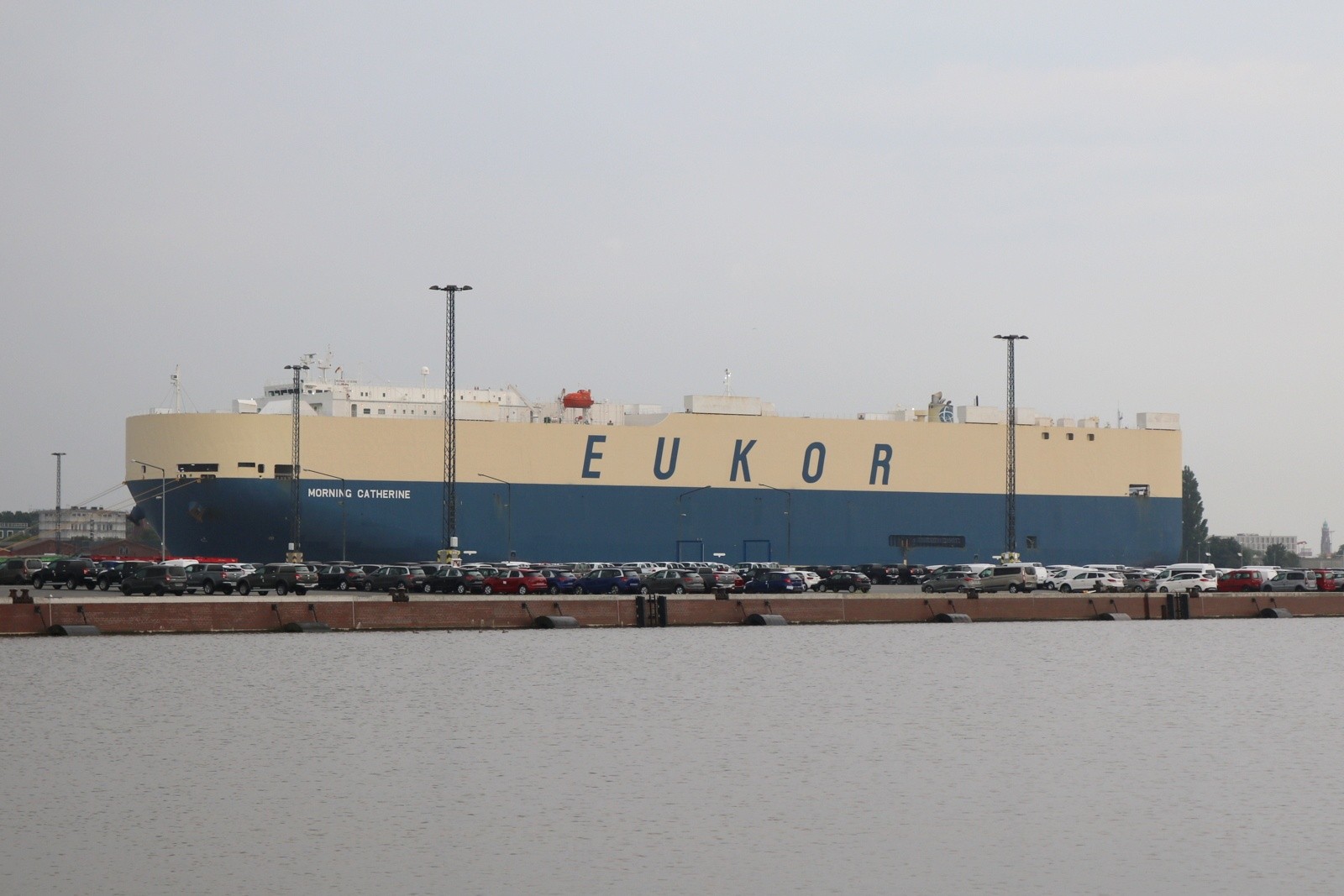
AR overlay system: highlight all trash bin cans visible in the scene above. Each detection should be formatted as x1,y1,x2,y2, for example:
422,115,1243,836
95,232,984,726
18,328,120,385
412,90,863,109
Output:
966,587,982,598
712,587,729,599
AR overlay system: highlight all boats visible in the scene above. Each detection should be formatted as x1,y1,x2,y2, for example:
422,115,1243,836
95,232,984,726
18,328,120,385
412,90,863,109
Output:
562,389,594,408
125,357,1184,568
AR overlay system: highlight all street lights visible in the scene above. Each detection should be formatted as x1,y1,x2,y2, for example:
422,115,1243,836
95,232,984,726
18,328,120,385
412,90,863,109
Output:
304,468,346,561
429,284,476,550
678,486,710,562
759,483,790,567
284,364,315,549
51,452,67,554
1207,542,1210,562
131,460,165,562
1198,542,1201,563
994,335,1029,564
478,474,510,563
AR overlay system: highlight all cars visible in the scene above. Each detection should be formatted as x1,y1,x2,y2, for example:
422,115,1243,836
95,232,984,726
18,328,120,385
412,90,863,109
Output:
0,558,1344,596
